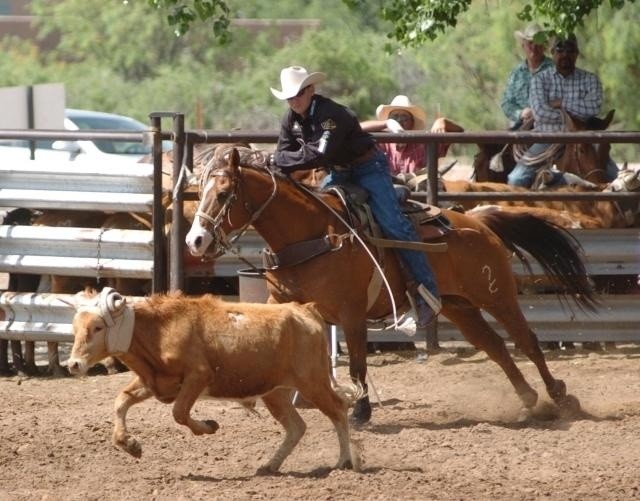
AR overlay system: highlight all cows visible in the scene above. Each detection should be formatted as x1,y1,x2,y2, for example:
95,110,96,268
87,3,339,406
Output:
58,284,372,478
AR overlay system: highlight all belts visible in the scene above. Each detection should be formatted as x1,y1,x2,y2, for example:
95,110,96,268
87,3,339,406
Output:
329,145,380,172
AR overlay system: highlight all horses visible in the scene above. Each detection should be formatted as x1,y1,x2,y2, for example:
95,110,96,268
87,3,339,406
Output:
369,108,640,353
1,160,344,378
185,146,604,434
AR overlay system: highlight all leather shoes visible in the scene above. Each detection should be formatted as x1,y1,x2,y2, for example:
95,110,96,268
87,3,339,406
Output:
415,302,438,330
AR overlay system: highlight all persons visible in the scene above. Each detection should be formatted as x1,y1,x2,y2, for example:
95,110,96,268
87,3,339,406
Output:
272,65,441,327
507,29,619,190
359,91,464,174
497,21,558,122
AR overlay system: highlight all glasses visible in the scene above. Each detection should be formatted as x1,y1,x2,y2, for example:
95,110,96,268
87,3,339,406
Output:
286,84,312,100
389,114,412,123
554,46,576,53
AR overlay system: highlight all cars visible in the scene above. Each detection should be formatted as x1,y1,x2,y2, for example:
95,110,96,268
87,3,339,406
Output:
1,108,175,178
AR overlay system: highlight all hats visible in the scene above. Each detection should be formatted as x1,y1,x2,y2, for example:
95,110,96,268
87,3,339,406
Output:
270,65,328,102
513,24,551,51
548,33,579,54
375,95,426,131
